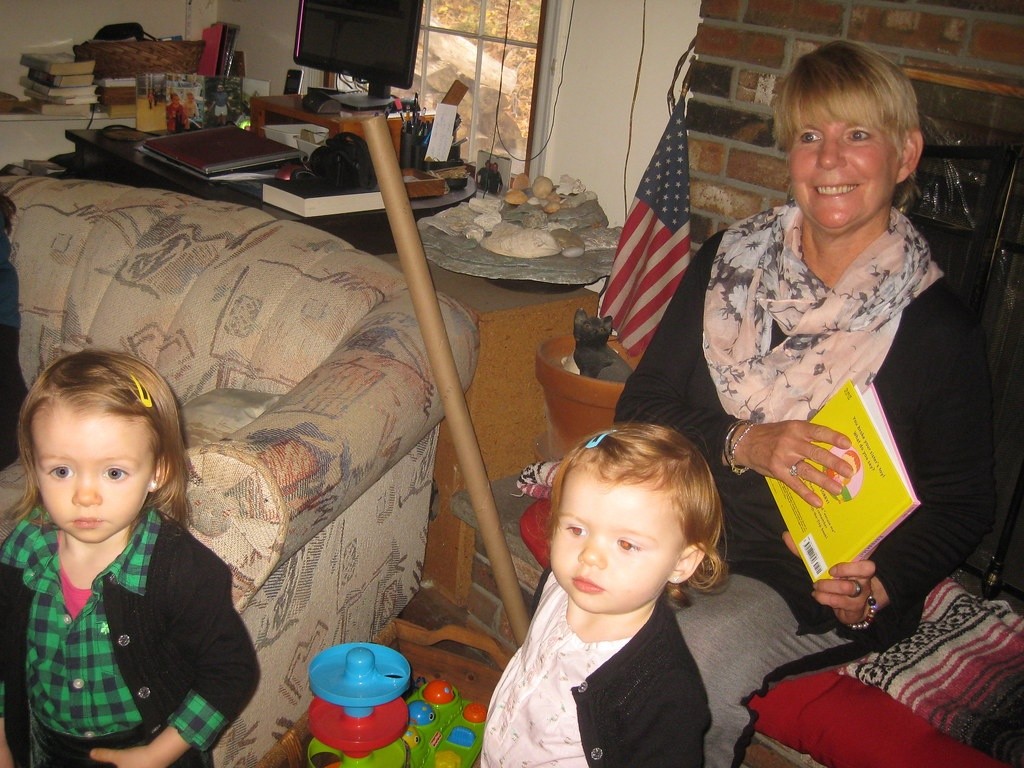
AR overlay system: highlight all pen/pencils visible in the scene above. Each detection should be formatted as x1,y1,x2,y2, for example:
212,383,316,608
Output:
424,113,462,144
403,93,434,136
395,97,405,122
454,137,468,146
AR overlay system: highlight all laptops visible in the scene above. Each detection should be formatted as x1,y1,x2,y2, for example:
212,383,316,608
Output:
143,125,310,177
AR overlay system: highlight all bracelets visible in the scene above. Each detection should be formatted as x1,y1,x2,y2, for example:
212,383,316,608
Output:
723,419,758,476
845,589,877,631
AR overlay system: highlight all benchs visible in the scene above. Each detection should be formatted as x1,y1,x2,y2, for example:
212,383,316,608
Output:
519,476,1024,768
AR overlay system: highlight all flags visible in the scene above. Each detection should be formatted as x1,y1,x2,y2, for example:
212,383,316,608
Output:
600,92,693,357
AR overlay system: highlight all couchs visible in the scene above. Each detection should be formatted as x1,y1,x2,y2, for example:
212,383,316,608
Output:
0,173,481,768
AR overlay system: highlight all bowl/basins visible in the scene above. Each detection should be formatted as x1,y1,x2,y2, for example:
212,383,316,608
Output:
534,334,640,444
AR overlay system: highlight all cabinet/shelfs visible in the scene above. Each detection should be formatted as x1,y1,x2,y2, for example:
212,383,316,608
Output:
380,254,607,609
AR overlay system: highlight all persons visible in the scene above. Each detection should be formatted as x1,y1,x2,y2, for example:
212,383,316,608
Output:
611,38,999,768
147,84,163,109
209,82,236,125
481,422,731,768
475,161,504,195
182,92,200,119
167,92,190,132
0,345,260,768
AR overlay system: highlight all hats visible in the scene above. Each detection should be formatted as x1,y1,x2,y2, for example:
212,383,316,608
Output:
485,160,491,167
217,85,224,92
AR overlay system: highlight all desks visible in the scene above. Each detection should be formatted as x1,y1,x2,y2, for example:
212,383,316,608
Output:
61,128,475,257
453,472,544,642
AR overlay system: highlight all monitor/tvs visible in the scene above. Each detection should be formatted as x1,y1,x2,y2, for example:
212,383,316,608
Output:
293,0,424,111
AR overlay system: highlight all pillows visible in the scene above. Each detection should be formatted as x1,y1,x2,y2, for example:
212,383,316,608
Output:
178,387,285,448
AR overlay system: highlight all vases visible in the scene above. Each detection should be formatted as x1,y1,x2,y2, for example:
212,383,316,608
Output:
535,333,643,460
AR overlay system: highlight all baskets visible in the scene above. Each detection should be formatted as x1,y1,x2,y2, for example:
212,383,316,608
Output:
0,91,18,113
73,40,206,78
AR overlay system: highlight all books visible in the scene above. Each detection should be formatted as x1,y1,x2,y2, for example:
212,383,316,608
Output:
262,180,386,218
763,379,921,583
197,22,240,78
135,124,309,180
166,73,205,133
203,76,241,127
241,77,270,120
93,78,136,118
19,51,101,115
135,72,166,132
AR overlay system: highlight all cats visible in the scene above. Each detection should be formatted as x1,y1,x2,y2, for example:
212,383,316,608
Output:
573,307,634,383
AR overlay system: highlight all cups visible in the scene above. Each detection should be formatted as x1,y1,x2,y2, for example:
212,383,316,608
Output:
401,125,426,169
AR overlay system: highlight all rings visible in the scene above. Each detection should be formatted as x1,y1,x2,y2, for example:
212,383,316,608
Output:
790,459,804,477
849,580,861,597
